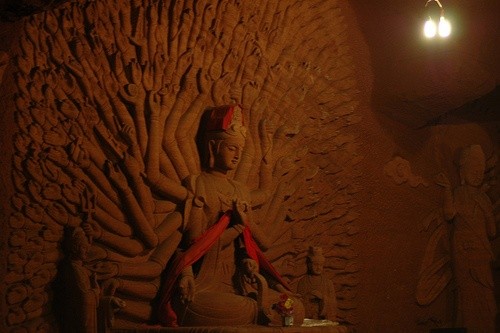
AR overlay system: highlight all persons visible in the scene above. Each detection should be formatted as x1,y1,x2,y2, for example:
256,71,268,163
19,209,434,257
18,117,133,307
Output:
2,1,377,333
435,145,500,333
